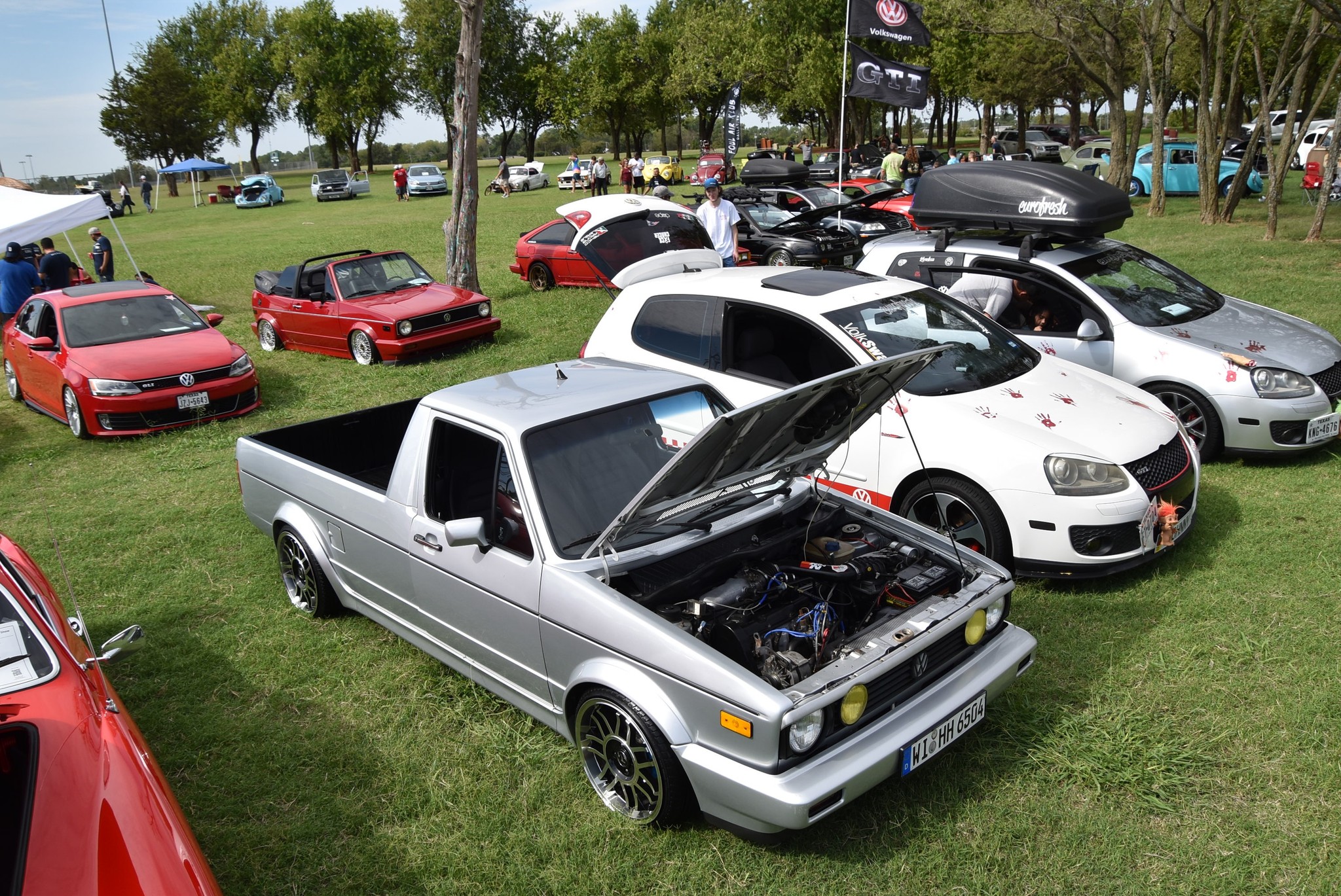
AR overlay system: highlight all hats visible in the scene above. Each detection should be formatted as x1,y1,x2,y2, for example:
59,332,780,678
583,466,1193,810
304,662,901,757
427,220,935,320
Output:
393,165,399,169
652,185,674,198
633,152,638,156
497,156,503,159
5,242,21,258
704,178,718,190
398,165,403,168
139,175,146,179
88,226,103,234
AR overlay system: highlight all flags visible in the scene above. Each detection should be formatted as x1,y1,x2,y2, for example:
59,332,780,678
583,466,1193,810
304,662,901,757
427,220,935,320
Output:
844,39,931,110
848,0,931,47
724,80,742,164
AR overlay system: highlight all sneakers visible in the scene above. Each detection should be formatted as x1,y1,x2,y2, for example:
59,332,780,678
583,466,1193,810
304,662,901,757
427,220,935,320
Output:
503,195,510,198
502,194,507,198
150,208,154,213
147,211,150,214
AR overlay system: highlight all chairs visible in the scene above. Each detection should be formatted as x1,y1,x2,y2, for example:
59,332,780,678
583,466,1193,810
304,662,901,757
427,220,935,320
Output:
217,185,235,203
803,333,846,382
1302,161,1324,208
348,264,382,298
734,318,798,387
306,268,326,299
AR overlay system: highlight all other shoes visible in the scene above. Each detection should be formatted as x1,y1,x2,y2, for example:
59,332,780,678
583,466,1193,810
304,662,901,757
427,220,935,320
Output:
397,196,409,202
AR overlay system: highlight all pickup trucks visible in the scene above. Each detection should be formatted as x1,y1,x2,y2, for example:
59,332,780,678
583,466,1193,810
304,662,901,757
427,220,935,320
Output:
235,343,1043,837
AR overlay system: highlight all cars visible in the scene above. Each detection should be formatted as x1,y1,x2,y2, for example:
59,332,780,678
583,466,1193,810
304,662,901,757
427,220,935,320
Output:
556,193,1201,583
803,108,1341,201
1,279,262,440
1102,137,1263,200
682,184,865,273
557,160,612,190
235,174,285,209
406,164,447,196
491,161,551,192
855,161,1341,463
825,178,934,232
509,217,756,293
311,169,370,202
740,149,915,255
689,153,737,186
642,156,685,185
251,250,501,367
0,520,225,896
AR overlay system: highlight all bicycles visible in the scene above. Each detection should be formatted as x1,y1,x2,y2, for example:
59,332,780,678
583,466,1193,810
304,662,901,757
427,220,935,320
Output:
484,179,513,196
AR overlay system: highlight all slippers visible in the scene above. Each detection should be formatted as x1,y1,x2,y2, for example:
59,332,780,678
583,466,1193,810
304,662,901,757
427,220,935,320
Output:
569,192,575,194
582,191,588,193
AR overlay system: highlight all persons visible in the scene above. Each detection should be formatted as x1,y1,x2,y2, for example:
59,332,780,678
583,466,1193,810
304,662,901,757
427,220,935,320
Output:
933,161,940,169
784,142,795,162
139,175,154,214
135,271,161,286
1014,302,1051,332
881,143,904,187
588,156,598,197
991,136,1001,160
21,242,43,271
37,237,74,291
393,165,409,202
0,242,43,326
849,143,865,168
71,262,96,287
118,180,135,214
885,136,891,149
653,185,670,201
945,271,1043,322
397,164,409,200
88,227,115,283
947,147,960,165
643,168,667,196
568,152,587,194
696,178,741,268
899,146,923,194
495,156,510,198
592,157,608,196
796,138,816,166
619,161,635,194
873,135,882,148
892,133,903,146
627,152,645,195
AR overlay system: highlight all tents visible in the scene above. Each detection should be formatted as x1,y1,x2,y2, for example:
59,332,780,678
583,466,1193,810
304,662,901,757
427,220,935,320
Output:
0,185,145,284
155,158,239,210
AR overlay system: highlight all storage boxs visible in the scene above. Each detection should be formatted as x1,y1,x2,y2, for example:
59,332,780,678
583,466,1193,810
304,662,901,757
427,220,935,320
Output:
907,159,1134,242
208,193,217,203
739,158,810,185
